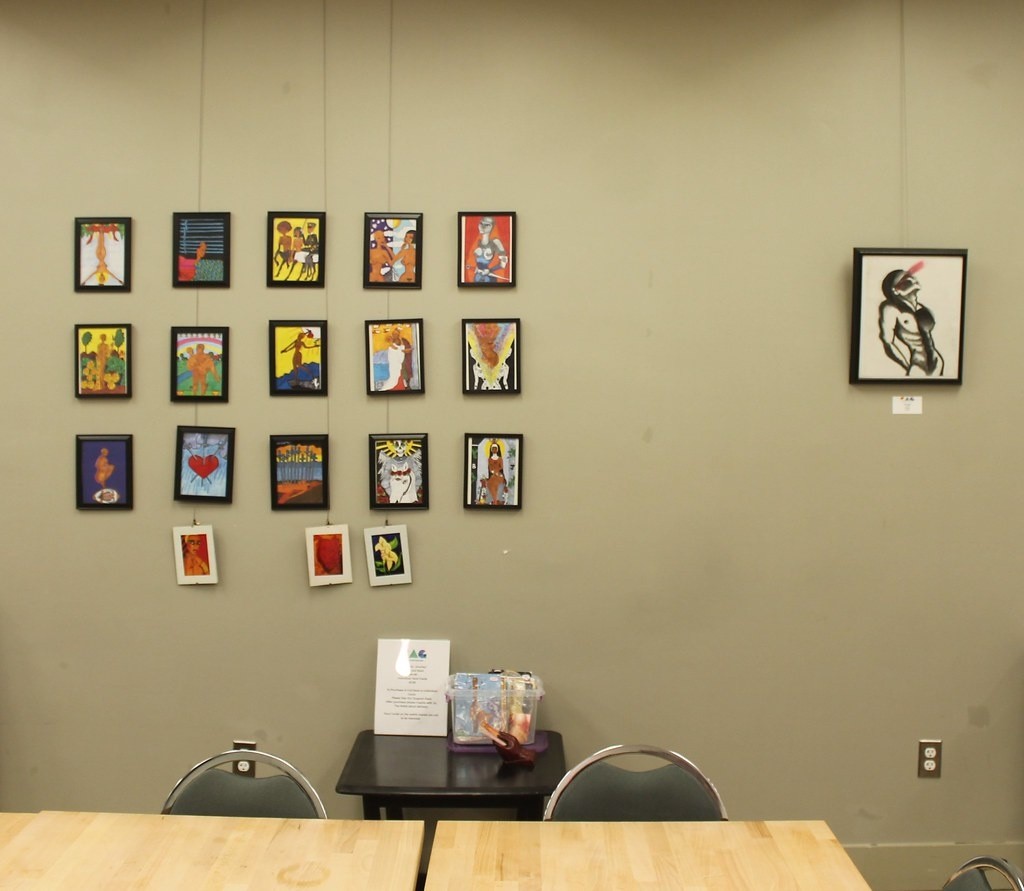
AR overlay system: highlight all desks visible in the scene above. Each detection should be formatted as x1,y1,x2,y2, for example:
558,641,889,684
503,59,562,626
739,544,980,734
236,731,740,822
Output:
0,808,423,891
335,729,566,821
423,819,871,891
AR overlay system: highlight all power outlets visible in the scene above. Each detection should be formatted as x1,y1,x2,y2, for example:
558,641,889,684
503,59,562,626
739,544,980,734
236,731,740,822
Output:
918,740,941,778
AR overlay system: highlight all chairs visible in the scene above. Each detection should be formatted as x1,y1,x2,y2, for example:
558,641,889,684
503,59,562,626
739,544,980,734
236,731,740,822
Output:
941,855,1024,891
159,739,327,818
542,741,728,821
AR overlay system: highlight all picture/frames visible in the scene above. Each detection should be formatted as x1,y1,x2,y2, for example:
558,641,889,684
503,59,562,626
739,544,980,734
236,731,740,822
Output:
74,324,133,399
305,523,353,587
268,319,328,397
74,211,515,292
365,318,425,396
75,434,134,509
171,325,229,402
369,433,429,511
173,525,218,585
461,319,520,394
175,425,235,502
363,525,412,586
268,433,329,510
849,248,968,387
463,432,522,510
